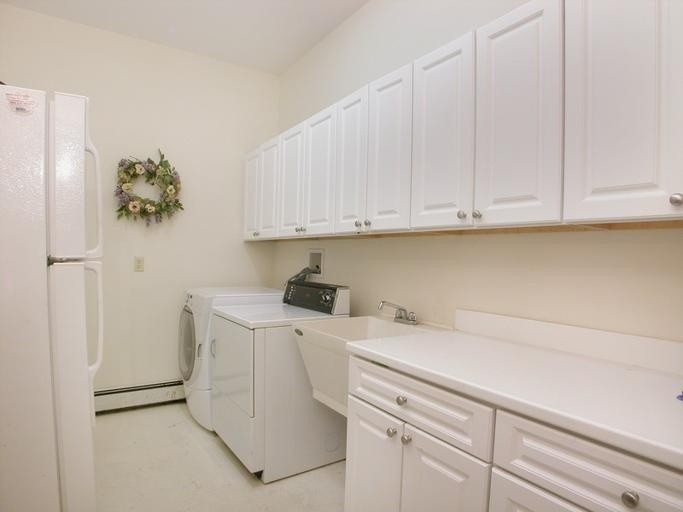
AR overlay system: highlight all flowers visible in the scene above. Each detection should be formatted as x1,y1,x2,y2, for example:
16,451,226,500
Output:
111,145,185,228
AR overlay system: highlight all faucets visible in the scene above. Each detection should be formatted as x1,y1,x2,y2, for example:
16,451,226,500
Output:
377,298,409,324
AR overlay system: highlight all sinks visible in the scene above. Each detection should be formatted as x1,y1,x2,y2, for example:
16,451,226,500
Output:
292,313,451,422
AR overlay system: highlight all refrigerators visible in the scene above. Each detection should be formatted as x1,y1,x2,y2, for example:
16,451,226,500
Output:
0,80,105,511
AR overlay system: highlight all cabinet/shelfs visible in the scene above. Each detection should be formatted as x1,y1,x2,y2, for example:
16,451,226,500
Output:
207,310,347,486
334,65,412,236
413,0,563,234
563,2,681,226
490,410,683,511
244,137,279,242
344,356,495,512
278,104,334,240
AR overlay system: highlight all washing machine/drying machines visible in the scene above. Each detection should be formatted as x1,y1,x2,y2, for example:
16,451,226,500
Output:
175,285,290,433
